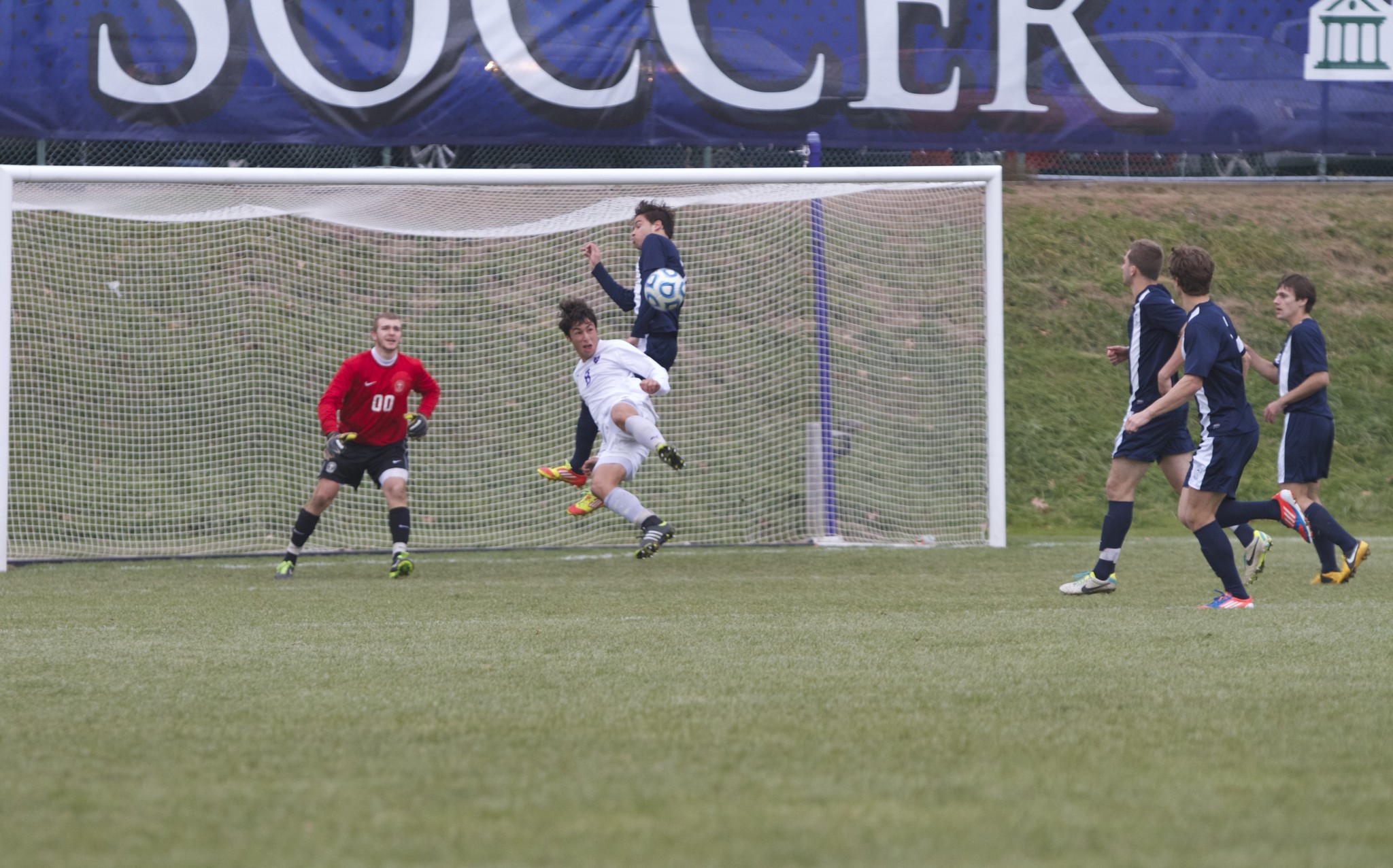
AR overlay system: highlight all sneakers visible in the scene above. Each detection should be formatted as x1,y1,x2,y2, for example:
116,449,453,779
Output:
635,522,675,560
388,552,414,579
537,460,588,488
1272,490,1313,544
275,560,295,579
1198,591,1253,609
1342,541,1371,578
567,489,606,516
1243,530,1273,586
1059,571,1117,596
658,443,687,471
1311,572,1349,586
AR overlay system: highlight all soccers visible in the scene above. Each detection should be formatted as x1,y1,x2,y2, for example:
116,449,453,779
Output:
644,267,686,311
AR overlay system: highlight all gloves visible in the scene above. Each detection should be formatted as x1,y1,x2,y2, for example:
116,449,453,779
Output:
404,411,428,439
323,431,357,461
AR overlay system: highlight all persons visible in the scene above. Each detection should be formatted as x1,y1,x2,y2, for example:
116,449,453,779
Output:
1059,240,1272,596
1245,273,1371,584
537,200,687,518
274,314,441,580
557,297,685,557
1125,246,1311,608
